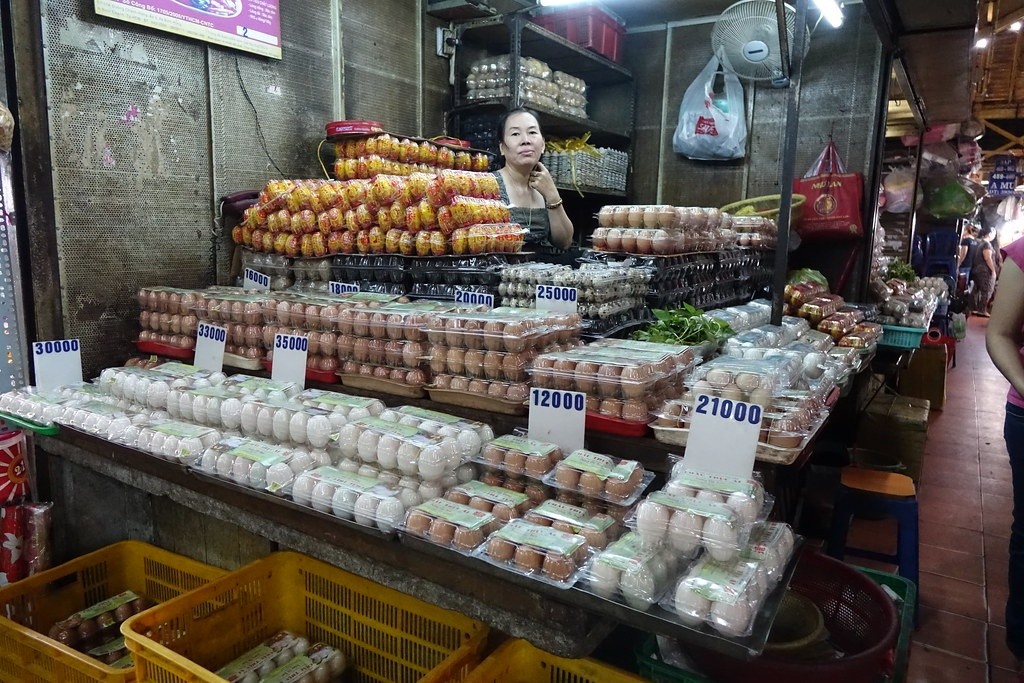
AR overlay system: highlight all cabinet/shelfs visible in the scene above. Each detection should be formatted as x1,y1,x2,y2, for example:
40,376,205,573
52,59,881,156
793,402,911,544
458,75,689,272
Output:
442,17,636,197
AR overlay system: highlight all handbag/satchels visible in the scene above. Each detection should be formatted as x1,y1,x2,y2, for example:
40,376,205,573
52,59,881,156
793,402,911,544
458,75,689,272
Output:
793,141,868,239
884,143,988,220
671,46,748,160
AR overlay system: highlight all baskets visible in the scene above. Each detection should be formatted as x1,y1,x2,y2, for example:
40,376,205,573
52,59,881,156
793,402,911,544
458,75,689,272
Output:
711,549,917,683
0,540,654,682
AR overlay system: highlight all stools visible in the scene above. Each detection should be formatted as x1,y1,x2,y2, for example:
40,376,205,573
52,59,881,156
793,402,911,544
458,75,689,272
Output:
825,466,918,626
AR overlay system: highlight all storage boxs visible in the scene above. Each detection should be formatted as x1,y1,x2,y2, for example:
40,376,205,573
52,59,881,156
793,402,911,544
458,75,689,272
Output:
0,539,916,683
529,0,628,66
857,393,930,486
896,343,948,412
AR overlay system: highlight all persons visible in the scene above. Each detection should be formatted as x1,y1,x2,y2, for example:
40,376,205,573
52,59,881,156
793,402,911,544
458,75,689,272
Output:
912,225,996,318
985,238,1024,666
491,106,574,264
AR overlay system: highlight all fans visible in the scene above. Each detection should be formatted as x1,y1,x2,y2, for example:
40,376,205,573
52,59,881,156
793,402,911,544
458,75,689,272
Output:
710,0,811,89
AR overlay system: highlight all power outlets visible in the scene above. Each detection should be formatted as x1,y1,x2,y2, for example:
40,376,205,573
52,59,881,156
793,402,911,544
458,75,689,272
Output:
436,26,454,58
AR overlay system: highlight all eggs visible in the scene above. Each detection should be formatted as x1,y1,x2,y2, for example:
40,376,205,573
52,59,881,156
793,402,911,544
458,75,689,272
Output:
0,256,947,644
233,134,776,251
467,56,590,119
50,597,347,681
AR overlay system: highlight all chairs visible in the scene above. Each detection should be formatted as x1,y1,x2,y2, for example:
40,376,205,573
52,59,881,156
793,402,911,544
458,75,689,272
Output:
910,231,959,297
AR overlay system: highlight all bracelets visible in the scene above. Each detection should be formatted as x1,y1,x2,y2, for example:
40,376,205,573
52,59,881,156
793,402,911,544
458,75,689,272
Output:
545,199,563,209
